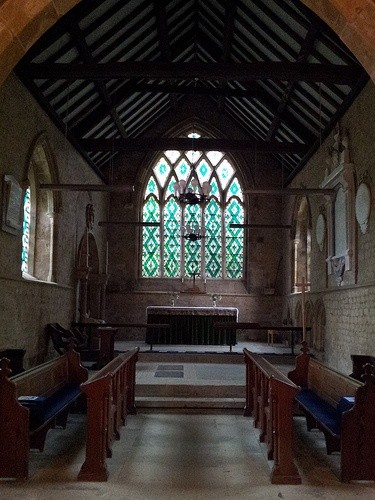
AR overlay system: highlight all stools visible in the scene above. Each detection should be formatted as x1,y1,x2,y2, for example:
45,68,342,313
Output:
267,329,294,347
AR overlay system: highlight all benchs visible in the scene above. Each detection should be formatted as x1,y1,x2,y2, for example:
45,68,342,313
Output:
283,340,374,488
0,335,92,485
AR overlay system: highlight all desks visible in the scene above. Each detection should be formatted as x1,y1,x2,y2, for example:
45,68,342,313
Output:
144,306,239,346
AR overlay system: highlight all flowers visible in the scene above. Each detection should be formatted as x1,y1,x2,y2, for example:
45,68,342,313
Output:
168,293,179,303
208,293,222,303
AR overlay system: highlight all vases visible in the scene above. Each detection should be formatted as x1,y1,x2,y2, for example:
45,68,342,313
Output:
170,299,175,307
212,301,218,308
